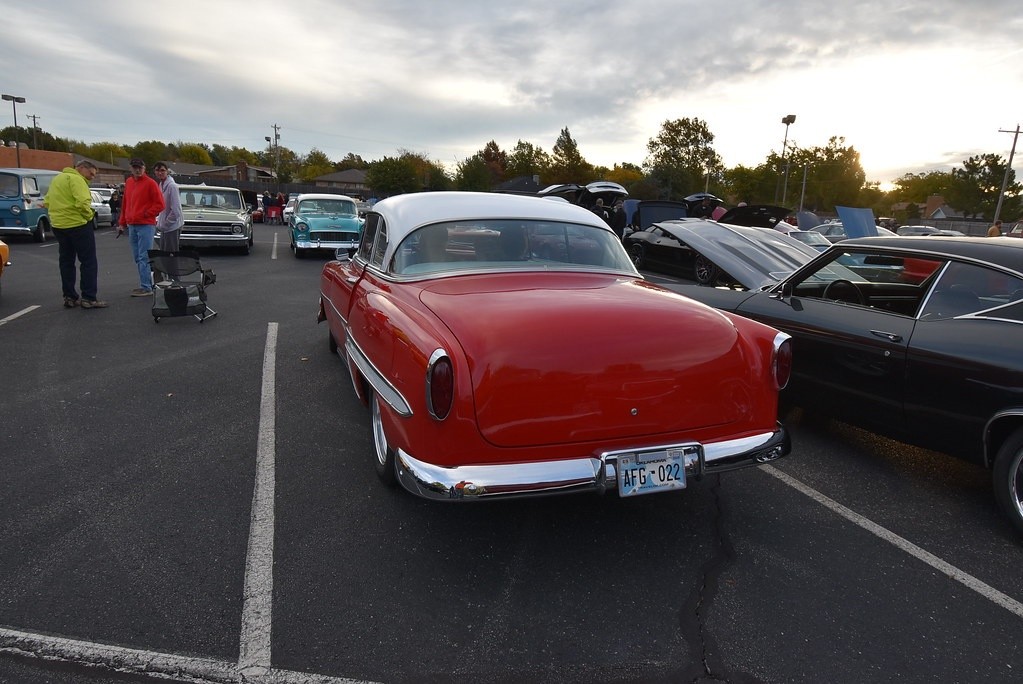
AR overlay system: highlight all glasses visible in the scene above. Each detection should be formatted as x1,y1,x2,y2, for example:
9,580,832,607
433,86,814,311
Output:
154,169,167,173
86,167,96,179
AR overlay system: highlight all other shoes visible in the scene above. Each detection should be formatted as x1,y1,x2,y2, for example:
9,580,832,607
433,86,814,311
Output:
64,297,81,306
80,298,109,307
131,288,153,296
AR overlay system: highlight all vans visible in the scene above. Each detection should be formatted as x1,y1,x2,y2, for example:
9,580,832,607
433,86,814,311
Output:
0,168,63,243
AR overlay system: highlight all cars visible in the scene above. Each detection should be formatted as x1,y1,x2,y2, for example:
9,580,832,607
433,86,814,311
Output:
316,191,792,503
89,188,117,229
283,192,373,259
154,184,264,256
536,181,1022,530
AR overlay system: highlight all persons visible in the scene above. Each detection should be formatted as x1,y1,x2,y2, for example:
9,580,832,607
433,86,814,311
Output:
106,191,120,228
988,220,1002,237
590,198,607,223
692,198,713,220
262,191,289,225
152,162,185,293
43,160,110,308
611,200,627,244
116,159,166,296
738,202,748,208
874,217,900,234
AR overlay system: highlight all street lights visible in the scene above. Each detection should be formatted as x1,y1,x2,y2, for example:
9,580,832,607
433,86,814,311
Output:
775,115,796,207
2,95,25,168
265,137,272,182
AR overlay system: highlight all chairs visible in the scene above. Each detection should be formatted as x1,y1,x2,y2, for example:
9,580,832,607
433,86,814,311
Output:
147,249,218,323
493,224,528,258
913,283,985,318
404,225,458,268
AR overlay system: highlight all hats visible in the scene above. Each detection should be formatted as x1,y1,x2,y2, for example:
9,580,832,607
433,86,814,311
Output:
129,159,145,167
